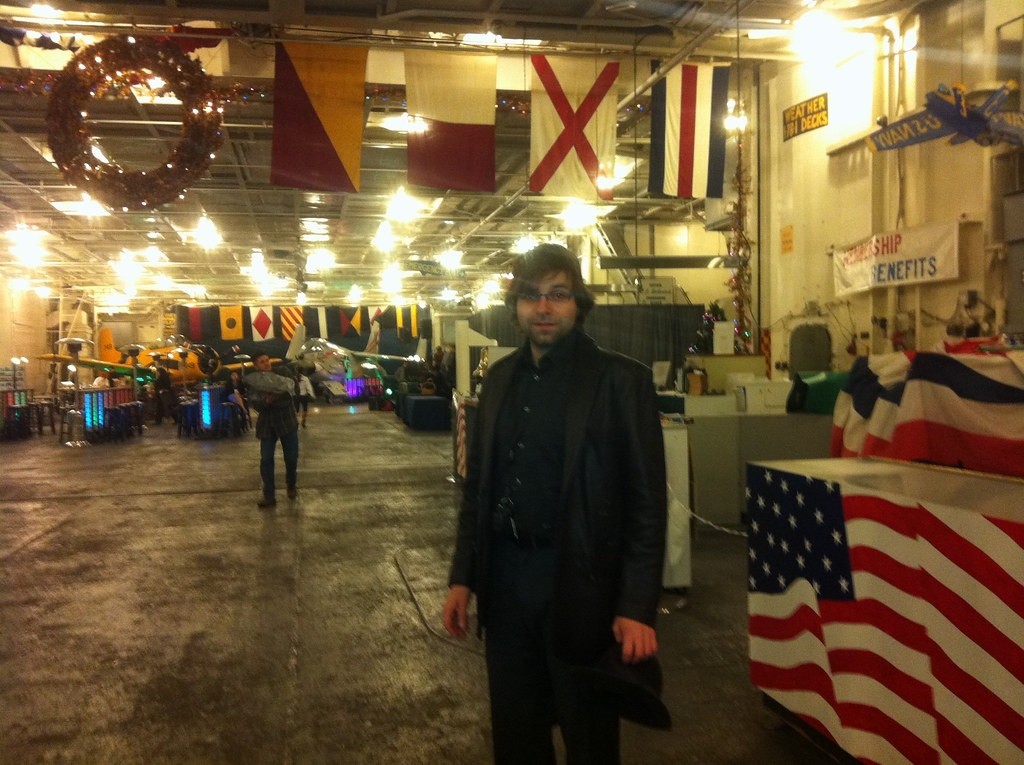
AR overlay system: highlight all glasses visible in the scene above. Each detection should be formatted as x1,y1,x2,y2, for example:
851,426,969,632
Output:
516,289,575,303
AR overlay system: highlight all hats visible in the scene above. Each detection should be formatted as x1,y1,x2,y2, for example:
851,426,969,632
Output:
570,643,673,732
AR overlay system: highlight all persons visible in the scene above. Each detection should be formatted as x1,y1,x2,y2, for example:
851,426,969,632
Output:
88,360,133,420
152,367,181,426
440,245,669,765
245,350,300,508
393,344,455,418
292,366,318,427
223,371,248,435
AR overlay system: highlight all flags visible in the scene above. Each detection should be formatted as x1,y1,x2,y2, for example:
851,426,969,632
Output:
173,303,429,348
270,40,369,194
647,59,731,199
528,53,619,202
402,47,497,192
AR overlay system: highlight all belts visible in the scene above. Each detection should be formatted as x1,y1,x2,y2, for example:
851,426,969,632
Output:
499,525,555,553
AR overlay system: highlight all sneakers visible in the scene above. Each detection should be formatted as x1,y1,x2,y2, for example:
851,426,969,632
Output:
256,498,275,507
286,488,296,498
